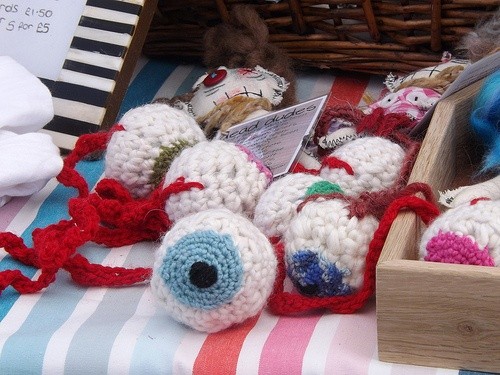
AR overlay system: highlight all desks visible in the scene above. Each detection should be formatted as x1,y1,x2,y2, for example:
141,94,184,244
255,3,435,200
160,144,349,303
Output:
1,56,500,375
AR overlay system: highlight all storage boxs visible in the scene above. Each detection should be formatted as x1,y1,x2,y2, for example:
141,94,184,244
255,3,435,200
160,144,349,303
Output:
142,0,500,79
376,69,500,375
